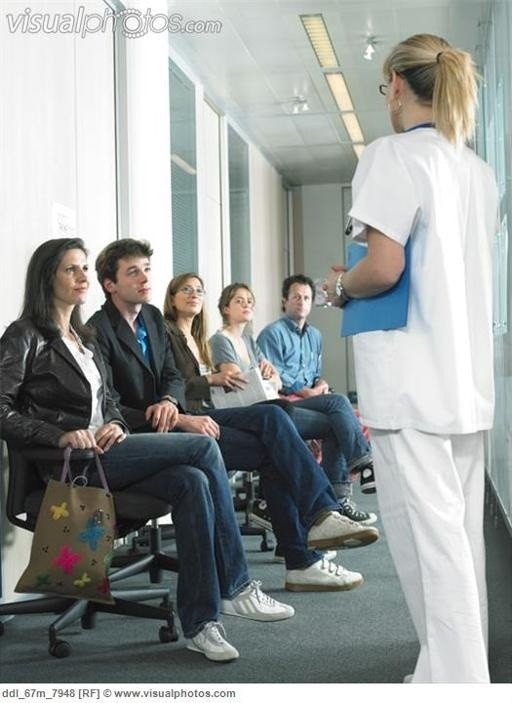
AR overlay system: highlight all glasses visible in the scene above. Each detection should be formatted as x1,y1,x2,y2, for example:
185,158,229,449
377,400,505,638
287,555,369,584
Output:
179,285,206,295
378,70,399,98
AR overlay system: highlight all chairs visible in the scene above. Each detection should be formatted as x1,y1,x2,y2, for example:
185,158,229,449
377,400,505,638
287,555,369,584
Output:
0,427,277,659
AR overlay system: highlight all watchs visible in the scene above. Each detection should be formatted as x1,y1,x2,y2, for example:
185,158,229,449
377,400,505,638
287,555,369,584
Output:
335,273,350,302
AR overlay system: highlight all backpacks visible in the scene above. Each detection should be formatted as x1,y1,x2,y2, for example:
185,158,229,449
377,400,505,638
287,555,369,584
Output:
10,440,123,609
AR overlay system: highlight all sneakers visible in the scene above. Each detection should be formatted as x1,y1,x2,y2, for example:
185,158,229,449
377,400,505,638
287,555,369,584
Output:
219,579,296,623
305,509,380,549
270,546,338,564
186,620,241,662
358,463,378,495
282,555,364,593
247,498,273,530
335,497,378,526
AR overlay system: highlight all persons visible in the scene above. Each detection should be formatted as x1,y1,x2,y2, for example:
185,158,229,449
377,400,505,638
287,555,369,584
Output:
1,237,295,662
161,272,333,562
325,34,501,684
86,238,378,593
258,275,329,400
208,280,378,524
